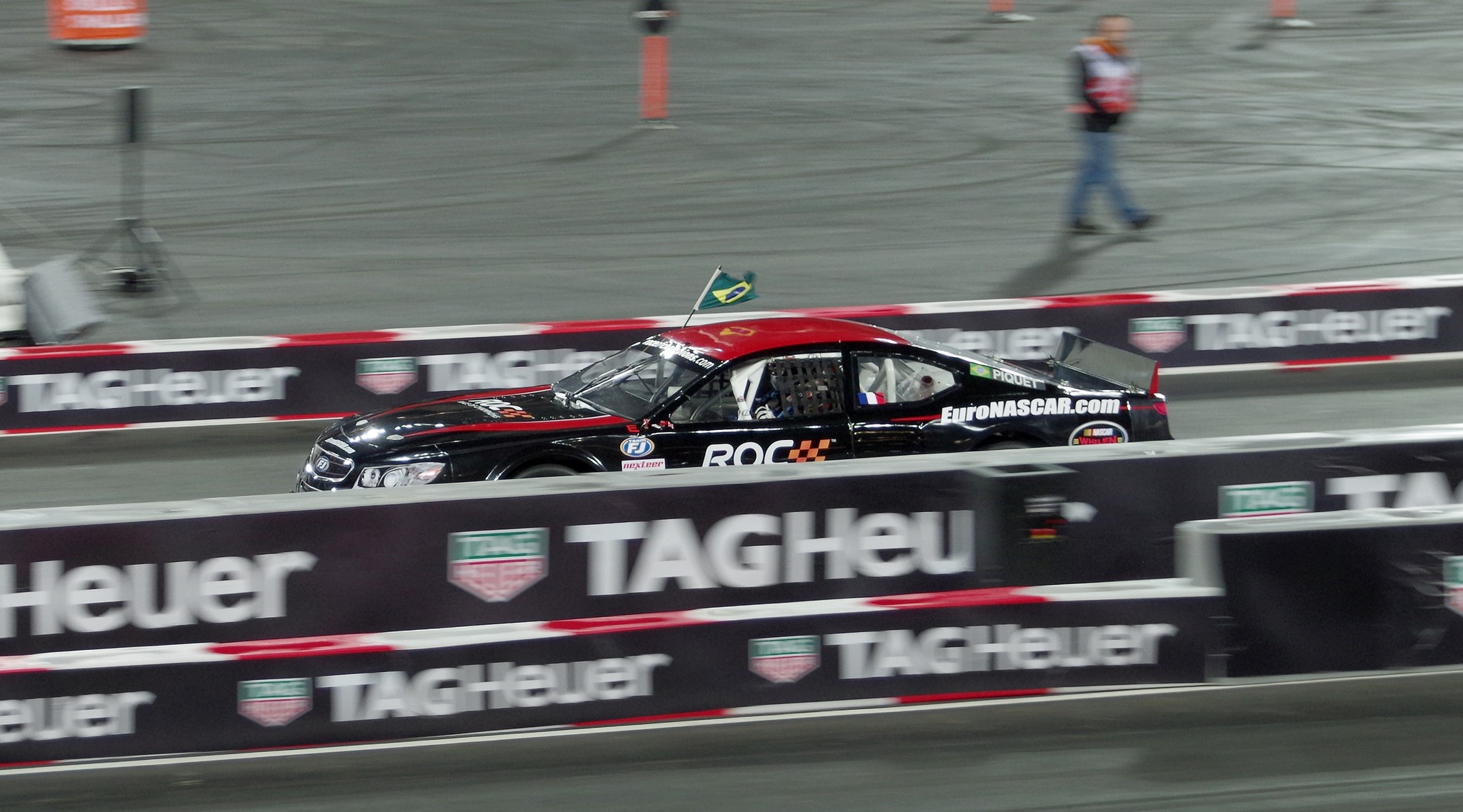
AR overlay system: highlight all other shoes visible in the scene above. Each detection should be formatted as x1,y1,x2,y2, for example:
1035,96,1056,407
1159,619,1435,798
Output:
1065,218,1104,233
1134,213,1154,229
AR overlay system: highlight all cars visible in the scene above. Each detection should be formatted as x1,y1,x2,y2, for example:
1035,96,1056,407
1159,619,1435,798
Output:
301,315,1169,495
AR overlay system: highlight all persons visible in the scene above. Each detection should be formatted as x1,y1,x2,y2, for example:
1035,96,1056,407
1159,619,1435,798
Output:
753,359,832,419
1060,14,1163,233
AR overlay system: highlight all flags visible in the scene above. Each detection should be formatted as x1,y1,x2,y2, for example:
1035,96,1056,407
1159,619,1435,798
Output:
693,268,759,311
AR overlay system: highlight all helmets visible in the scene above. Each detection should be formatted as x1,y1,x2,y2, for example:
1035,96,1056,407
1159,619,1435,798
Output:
768,361,810,391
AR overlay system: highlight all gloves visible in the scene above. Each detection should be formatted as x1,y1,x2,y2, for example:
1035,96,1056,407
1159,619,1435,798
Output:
754,404,779,420
